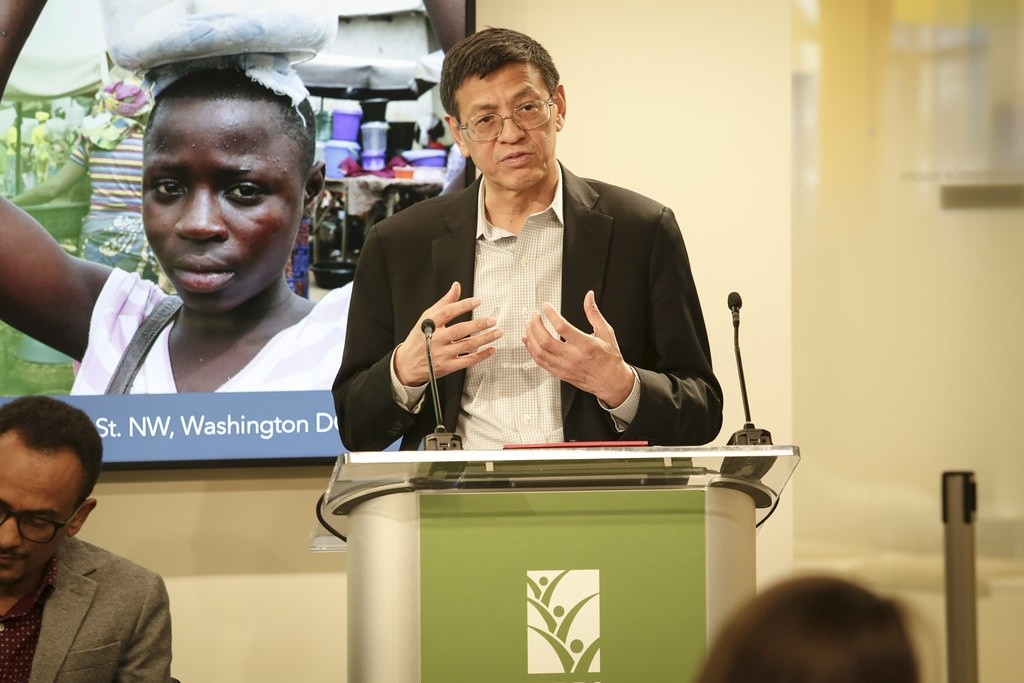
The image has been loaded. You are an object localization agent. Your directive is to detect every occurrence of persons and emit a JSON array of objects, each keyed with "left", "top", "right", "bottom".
[
  {"left": 0, "top": 395, "right": 173, "bottom": 683},
  {"left": 332, "top": 28, "right": 724, "bottom": 452},
  {"left": 0, "top": 0, "right": 353, "bottom": 396},
  {"left": 694, "top": 573, "right": 931, "bottom": 683}
]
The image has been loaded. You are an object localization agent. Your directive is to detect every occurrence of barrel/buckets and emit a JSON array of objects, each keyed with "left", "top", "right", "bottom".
[
  {"left": 324, "top": 139, "right": 361, "bottom": 179},
  {"left": 331, "top": 100, "right": 363, "bottom": 142},
  {"left": 395, "top": 167, "right": 415, "bottom": 181},
  {"left": 360, "top": 121, "right": 391, "bottom": 152},
  {"left": 361, "top": 152, "right": 386, "bottom": 172}
]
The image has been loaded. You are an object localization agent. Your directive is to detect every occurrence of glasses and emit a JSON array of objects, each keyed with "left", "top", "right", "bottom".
[
  {"left": 458, "top": 96, "right": 554, "bottom": 142},
  {"left": 0, "top": 495, "right": 86, "bottom": 544}
]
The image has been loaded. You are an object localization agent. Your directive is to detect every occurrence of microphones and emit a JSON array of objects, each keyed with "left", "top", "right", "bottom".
[
  {"left": 721, "top": 292, "right": 777, "bottom": 479},
  {"left": 418, "top": 319, "right": 467, "bottom": 484}
]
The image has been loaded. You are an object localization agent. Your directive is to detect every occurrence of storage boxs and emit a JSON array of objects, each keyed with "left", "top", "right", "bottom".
[{"left": 323, "top": 102, "right": 446, "bottom": 179}]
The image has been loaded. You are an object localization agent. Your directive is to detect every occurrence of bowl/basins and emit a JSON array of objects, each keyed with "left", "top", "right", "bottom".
[{"left": 310, "top": 262, "right": 356, "bottom": 289}]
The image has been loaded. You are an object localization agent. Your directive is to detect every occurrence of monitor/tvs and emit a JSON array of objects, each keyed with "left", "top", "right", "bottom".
[{"left": 0, "top": 0, "right": 476, "bottom": 469}]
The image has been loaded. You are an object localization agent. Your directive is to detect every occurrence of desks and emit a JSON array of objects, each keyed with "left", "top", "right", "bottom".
[{"left": 315, "top": 178, "right": 443, "bottom": 268}]
